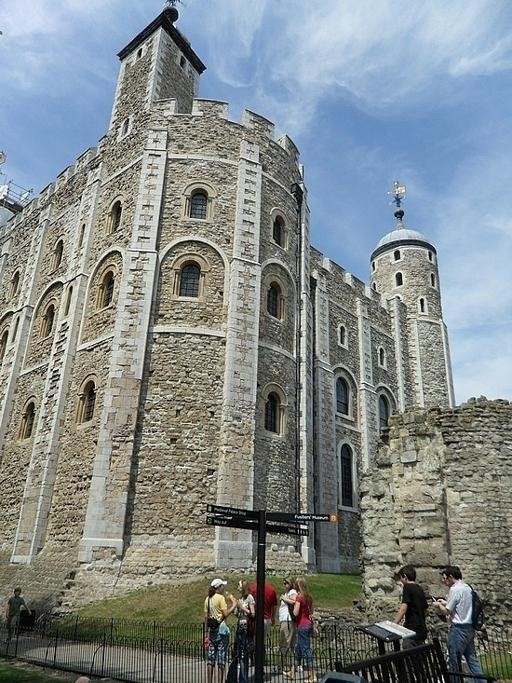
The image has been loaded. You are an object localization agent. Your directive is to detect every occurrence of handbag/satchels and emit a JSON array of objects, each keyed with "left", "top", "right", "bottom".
[
  {"left": 305, "top": 616, "right": 320, "bottom": 639},
  {"left": 246, "top": 608, "right": 256, "bottom": 636},
  {"left": 207, "top": 596, "right": 225, "bottom": 630},
  {"left": 236, "top": 608, "right": 249, "bottom": 635},
  {"left": 201, "top": 637, "right": 209, "bottom": 651},
  {"left": 217, "top": 622, "right": 231, "bottom": 638},
  {"left": 286, "top": 595, "right": 298, "bottom": 623}
]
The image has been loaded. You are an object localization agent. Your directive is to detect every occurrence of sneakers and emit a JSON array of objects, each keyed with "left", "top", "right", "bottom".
[
  {"left": 282, "top": 669, "right": 296, "bottom": 680},
  {"left": 304, "top": 676, "right": 318, "bottom": 683}
]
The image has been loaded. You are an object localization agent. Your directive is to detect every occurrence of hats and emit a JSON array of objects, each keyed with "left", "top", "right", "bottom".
[
  {"left": 283, "top": 576, "right": 294, "bottom": 587},
  {"left": 210, "top": 578, "right": 228, "bottom": 588}
]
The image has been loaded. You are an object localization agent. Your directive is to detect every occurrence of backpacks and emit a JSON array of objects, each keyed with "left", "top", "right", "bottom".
[{"left": 466, "top": 582, "right": 486, "bottom": 632}]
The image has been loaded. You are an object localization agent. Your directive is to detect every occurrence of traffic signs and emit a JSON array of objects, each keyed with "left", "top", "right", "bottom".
[{"left": 206, "top": 503, "right": 336, "bottom": 535}]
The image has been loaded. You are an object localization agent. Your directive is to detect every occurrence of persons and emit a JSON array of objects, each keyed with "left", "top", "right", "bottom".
[
  {"left": 436, "top": 570, "right": 453, "bottom": 637},
  {"left": 226, "top": 578, "right": 254, "bottom": 666},
  {"left": 249, "top": 573, "right": 276, "bottom": 665},
  {"left": 393, "top": 566, "right": 426, "bottom": 661},
  {"left": 284, "top": 576, "right": 318, "bottom": 682},
  {"left": 274, "top": 576, "right": 303, "bottom": 673},
  {"left": 432, "top": 565, "right": 487, "bottom": 682},
  {"left": 202, "top": 577, "right": 238, "bottom": 682},
  {"left": 7, "top": 587, "right": 34, "bottom": 640}
]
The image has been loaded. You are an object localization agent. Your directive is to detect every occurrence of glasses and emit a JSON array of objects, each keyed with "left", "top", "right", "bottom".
[{"left": 284, "top": 582, "right": 288, "bottom": 585}]
